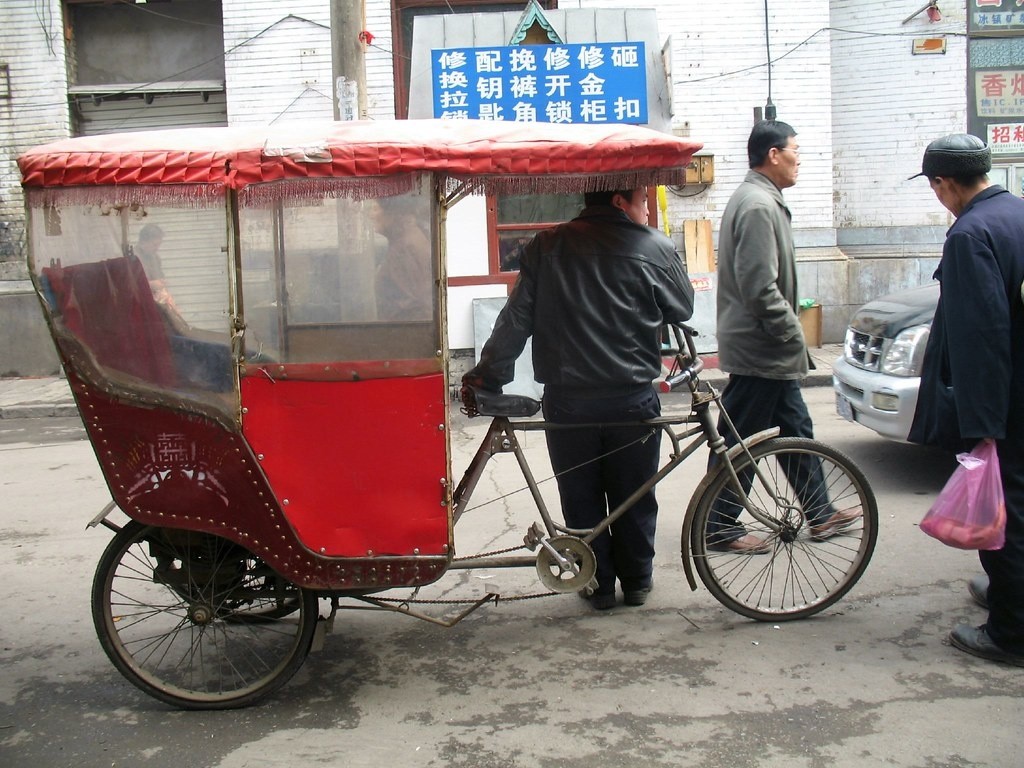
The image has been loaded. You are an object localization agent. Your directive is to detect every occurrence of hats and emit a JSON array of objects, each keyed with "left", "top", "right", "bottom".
[{"left": 906, "top": 133, "right": 992, "bottom": 182}]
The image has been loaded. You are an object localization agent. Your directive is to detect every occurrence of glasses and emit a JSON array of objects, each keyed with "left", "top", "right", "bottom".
[{"left": 778, "top": 147, "right": 803, "bottom": 159}]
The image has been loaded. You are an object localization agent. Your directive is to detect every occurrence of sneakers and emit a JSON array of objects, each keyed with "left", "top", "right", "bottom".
[
  {"left": 620, "top": 577, "right": 655, "bottom": 608},
  {"left": 576, "top": 578, "right": 618, "bottom": 614}
]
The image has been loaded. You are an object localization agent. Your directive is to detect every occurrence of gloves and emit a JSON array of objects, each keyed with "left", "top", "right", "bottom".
[{"left": 461, "top": 364, "right": 503, "bottom": 398}]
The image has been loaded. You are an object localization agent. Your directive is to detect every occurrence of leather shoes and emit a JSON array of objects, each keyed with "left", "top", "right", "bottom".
[
  {"left": 967, "top": 573, "right": 995, "bottom": 610},
  {"left": 703, "top": 531, "right": 774, "bottom": 555},
  {"left": 808, "top": 506, "right": 865, "bottom": 541},
  {"left": 947, "top": 623, "right": 1023, "bottom": 668}
]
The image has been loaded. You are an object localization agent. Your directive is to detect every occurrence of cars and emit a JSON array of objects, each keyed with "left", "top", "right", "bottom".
[{"left": 830, "top": 280, "right": 945, "bottom": 450}]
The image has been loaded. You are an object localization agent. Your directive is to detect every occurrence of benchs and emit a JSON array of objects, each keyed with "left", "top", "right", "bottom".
[{"left": 43, "top": 258, "right": 279, "bottom": 416}]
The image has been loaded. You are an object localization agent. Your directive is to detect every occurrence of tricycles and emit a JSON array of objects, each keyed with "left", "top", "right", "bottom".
[{"left": 13, "top": 117, "right": 881, "bottom": 710}]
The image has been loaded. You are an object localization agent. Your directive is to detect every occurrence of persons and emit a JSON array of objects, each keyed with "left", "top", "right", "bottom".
[
  {"left": 130, "top": 225, "right": 167, "bottom": 293},
  {"left": 906, "top": 135, "right": 1024, "bottom": 668},
  {"left": 462, "top": 180, "right": 695, "bottom": 609},
  {"left": 364, "top": 190, "right": 434, "bottom": 322},
  {"left": 705, "top": 121, "right": 864, "bottom": 554}
]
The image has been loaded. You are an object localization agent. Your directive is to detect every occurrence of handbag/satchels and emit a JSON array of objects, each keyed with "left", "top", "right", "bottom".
[{"left": 918, "top": 436, "right": 1011, "bottom": 551}]
